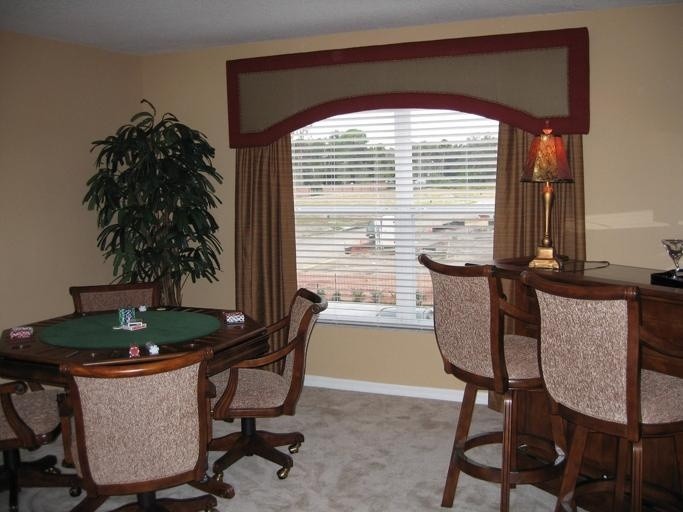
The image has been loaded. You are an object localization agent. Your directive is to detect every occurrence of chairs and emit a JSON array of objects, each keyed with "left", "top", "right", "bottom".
[
  {"left": 68, "top": 283, "right": 162, "bottom": 314},
  {"left": 208, "top": 287, "right": 327, "bottom": 482},
  {"left": 423, "top": 255, "right": 568, "bottom": 511},
  {"left": 1, "top": 381, "right": 82, "bottom": 511},
  {"left": 518, "top": 270, "right": 681, "bottom": 512},
  {"left": 55, "top": 348, "right": 235, "bottom": 512}
]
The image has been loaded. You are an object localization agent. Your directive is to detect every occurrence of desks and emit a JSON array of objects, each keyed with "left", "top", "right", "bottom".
[
  {"left": 1, "top": 307, "right": 267, "bottom": 390},
  {"left": 464, "top": 257, "right": 683, "bottom": 492}
]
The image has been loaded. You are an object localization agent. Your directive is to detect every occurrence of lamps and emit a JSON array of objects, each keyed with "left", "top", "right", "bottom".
[{"left": 520, "top": 118, "right": 576, "bottom": 271}]
